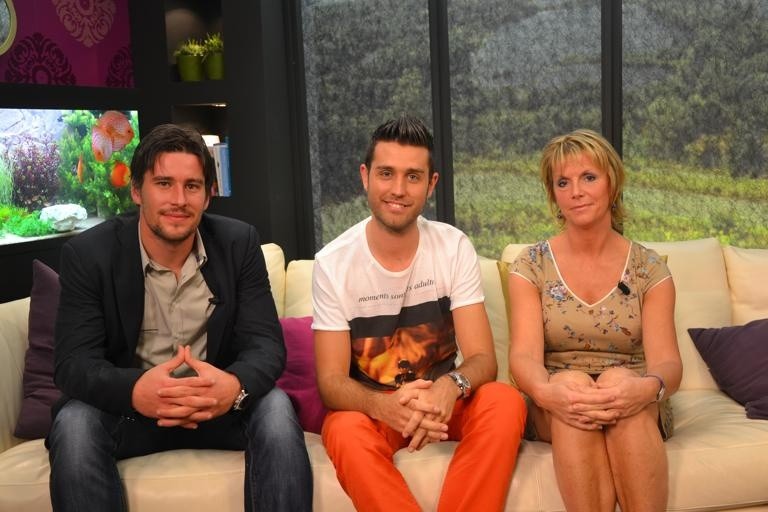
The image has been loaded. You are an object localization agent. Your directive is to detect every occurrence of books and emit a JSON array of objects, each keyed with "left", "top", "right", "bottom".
[{"left": 212, "top": 143, "right": 231, "bottom": 198}]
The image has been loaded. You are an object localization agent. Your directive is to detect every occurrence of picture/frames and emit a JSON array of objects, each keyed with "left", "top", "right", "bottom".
[{"left": 0, "top": 0, "right": 18, "bottom": 56}]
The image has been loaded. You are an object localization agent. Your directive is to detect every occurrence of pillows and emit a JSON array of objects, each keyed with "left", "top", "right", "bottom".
[
  {"left": 9, "top": 262, "right": 62, "bottom": 439},
  {"left": 285, "top": 253, "right": 509, "bottom": 385},
  {"left": 688, "top": 316, "right": 766, "bottom": 420},
  {"left": 279, "top": 317, "right": 322, "bottom": 435},
  {"left": 502, "top": 239, "right": 733, "bottom": 389},
  {"left": 261, "top": 242, "right": 285, "bottom": 316},
  {"left": 723, "top": 246, "right": 768, "bottom": 326}
]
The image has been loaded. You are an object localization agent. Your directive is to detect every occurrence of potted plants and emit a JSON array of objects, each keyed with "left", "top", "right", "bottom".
[
  {"left": 174, "top": 38, "right": 203, "bottom": 82},
  {"left": 201, "top": 30, "right": 225, "bottom": 82}
]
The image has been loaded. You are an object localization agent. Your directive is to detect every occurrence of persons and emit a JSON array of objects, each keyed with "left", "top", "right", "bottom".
[
  {"left": 311, "top": 115, "right": 529, "bottom": 512},
  {"left": 44, "top": 124, "right": 313, "bottom": 512},
  {"left": 507, "top": 130, "right": 683, "bottom": 512}
]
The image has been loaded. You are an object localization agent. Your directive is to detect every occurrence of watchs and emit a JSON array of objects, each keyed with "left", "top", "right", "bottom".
[
  {"left": 233, "top": 388, "right": 249, "bottom": 411},
  {"left": 645, "top": 374, "right": 665, "bottom": 406},
  {"left": 447, "top": 372, "right": 471, "bottom": 400}
]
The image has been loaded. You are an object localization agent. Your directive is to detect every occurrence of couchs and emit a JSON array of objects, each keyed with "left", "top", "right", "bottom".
[{"left": 0, "top": 237, "right": 768, "bottom": 512}]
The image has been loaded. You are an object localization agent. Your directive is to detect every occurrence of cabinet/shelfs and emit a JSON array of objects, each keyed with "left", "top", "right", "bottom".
[{"left": 0, "top": 236, "right": 71, "bottom": 303}]
